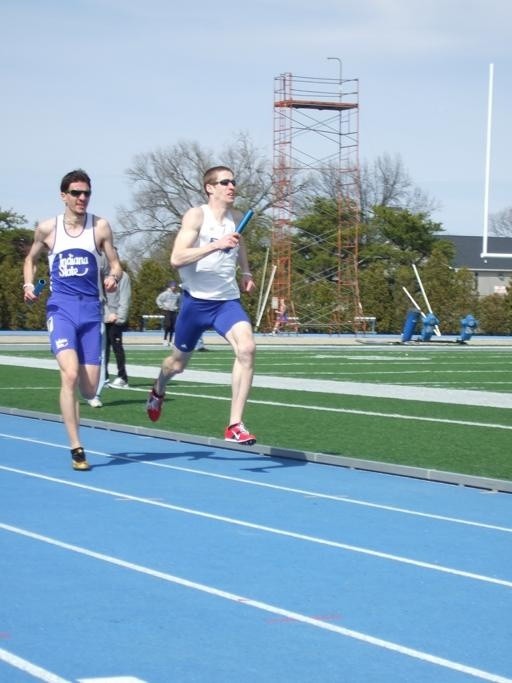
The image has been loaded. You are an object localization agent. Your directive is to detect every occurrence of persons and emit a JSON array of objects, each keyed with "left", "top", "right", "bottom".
[
  {"left": 99, "top": 246, "right": 132, "bottom": 390},
  {"left": 146, "top": 165, "right": 259, "bottom": 446},
  {"left": 22, "top": 167, "right": 128, "bottom": 470},
  {"left": 156, "top": 280, "right": 181, "bottom": 346},
  {"left": 271, "top": 298, "right": 298, "bottom": 334}
]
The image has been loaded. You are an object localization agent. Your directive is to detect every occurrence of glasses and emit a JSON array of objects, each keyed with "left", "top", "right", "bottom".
[
  {"left": 212, "top": 180, "right": 236, "bottom": 187},
  {"left": 66, "top": 191, "right": 90, "bottom": 197}
]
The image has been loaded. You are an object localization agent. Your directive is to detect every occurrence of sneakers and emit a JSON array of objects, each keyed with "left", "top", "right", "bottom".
[
  {"left": 87, "top": 398, "right": 103, "bottom": 407},
  {"left": 147, "top": 383, "right": 166, "bottom": 421},
  {"left": 225, "top": 422, "right": 256, "bottom": 445},
  {"left": 108, "top": 377, "right": 128, "bottom": 389},
  {"left": 71, "top": 447, "right": 89, "bottom": 470}
]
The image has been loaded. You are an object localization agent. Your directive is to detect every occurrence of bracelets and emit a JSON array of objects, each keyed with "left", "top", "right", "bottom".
[
  {"left": 23, "top": 282, "right": 35, "bottom": 289},
  {"left": 107, "top": 274, "right": 120, "bottom": 283},
  {"left": 239, "top": 271, "right": 254, "bottom": 278}
]
